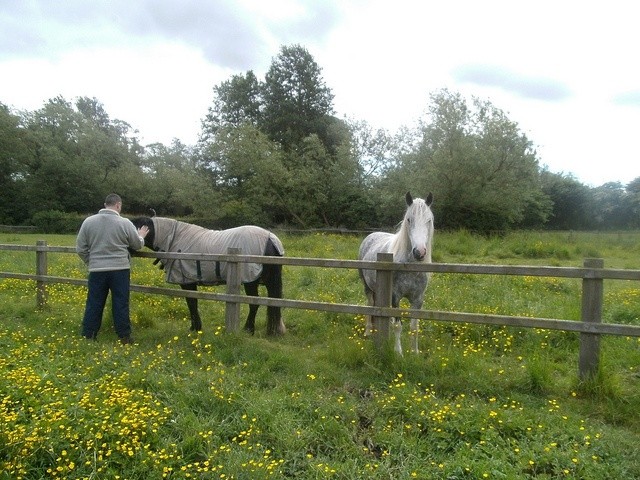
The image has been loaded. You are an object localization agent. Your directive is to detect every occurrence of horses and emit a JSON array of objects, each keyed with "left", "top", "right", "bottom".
[
  {"left": 357, "top": 192, "right": 435, "bottom": 357},
  {"left": 129, "top": 215, "right": 286, "bottom": 337}
]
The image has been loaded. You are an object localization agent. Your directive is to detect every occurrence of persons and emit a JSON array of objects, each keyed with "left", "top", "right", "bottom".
[{"left": 76, "top": 192, "right": 150, "bottom": 344}]
